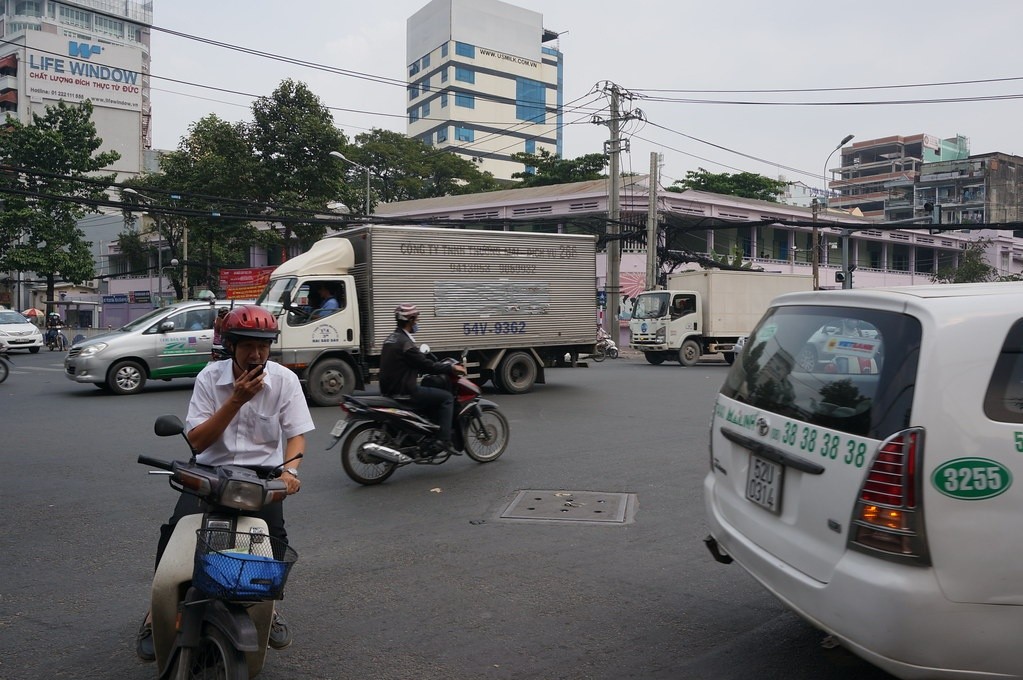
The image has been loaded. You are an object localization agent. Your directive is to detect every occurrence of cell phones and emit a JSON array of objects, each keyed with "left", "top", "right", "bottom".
[{"left": 248, "top": 362, "right": 264, "bottom": 382}]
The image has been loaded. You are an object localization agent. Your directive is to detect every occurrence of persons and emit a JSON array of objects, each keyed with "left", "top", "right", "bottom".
[
  {"left": 187, "top": 315, "right": 203, "bottom": 330},
  {"left": 379, "top": 304, "right": 468, "bottom": 456},
  {"left": 213, "top": 307, "right": 229, "bottom": 345},
  {"left": 299, "top": 283, "right": 338, "bottom": 319},
  {"left": 46, "top": 315, "right": 68, "bottom": 350},
  {"left": 597, "top": 323, "right": 609, "bottom": 341},
  {"left": 136, "top": 305, "right": 313, "bottom": 659}
]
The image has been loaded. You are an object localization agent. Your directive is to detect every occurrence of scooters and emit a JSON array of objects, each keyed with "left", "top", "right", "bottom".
[
  {"left": 590, "top": 331, "right": 620, "bottom": 361},
  {"left": 45, "top": 325, "right": 66, "bottom": 351},
  {"left": 0, "top": 348, "right": 15, "bottom": 383}
]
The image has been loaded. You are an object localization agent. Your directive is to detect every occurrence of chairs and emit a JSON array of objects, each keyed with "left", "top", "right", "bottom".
[
  {"left": 333, "top": 283, "right": 345, "bottom": 308},
  {"left": 685, "top": 300, "right": 695, "bottom": 310}
]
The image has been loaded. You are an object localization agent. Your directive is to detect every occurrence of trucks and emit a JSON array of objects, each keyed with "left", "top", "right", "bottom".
[
  {"left": 629, "top": 269, "right": 815, "bottom": 368},
  {"left": 256, "top": 225, "right": 601, "bottom": 408}
]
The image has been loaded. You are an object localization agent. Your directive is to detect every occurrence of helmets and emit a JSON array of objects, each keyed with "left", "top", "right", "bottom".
[
  {"left": 49, "top": 312, "right": 54, "bottom": 315},
  {"left": 221, "top": 305, "right": 280, "bottom": 338},
  {"left": 54, "top": 313, "right": 60, "bottom": 317},
  {"left": 395, "top": 303, "right": 420, "bottom": 320}
]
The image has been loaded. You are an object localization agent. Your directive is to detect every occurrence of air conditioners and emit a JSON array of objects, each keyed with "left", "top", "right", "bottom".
[
  {"left": 76, "top": 280, "right": 86, "bottom": 286},
  {"left": 24, "top": 277, "right": 34, "bottom": 285},
  {"left": 87, "top": 281, "right": 98, "bottom": 288}
]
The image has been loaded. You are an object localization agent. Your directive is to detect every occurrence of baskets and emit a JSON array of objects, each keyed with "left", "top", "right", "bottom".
[{"left": 192, "top": 529, "right": 298, "bottom": 602}]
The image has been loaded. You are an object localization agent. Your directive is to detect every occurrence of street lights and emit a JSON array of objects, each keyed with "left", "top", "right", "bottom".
[
  {"left": 122, "top": 186, "right": 163, "bottom": 305},
  {"left": 822, "top": 135, "right": 856, "bottom": 208},
  {"left": 329, "top": 151, "right": 370, "bottom": 214}
]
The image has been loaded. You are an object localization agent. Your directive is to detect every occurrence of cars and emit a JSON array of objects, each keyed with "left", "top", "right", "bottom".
[
  {"left": 62, "top": 291, "right": 298, "bottom": 395},
  {"left": 0, "top": 309, "right": 44, "bottom": 354},
  {"left": 701, "top": 280, "right": 1023, "bottom": 680}
]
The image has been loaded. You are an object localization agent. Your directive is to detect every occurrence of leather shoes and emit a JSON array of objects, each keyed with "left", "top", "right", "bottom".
[{"left": 435, "top": 441, "right": 463, "bottom": 455}]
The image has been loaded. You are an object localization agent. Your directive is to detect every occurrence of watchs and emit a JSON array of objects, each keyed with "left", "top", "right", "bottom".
[{"left": 283, "top": 468, "right": 298, "bottom": 477}]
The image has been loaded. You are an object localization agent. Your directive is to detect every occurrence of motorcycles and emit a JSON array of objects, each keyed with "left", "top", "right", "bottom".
[
  {"left": 322, "top": 341, "right": 512, "bottom": 484},
  {"left": 133, "top": 413, "right": 302, "bottom": 680}
]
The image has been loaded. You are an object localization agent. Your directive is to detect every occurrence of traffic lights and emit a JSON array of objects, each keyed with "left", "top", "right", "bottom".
[{"left": 834, "top": 270, "right": 847, "bottom": 282}]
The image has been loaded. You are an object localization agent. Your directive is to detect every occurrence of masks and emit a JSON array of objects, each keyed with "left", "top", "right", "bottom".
[
  {"left": 411, "top": 322, "right": 418, "bottom": 334},
  {"left": 54, "top": 316, "right": 57, "bottom": 319}
]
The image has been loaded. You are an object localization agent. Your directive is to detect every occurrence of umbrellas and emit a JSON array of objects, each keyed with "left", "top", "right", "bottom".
[{"left": 21, "top": 307, "right": 44, "bottom": 318}]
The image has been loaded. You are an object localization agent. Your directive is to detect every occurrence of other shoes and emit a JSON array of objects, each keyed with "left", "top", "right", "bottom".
[
  {"left": 268, "top": 608, "right": 292, "bottom": 650},
  {"left": 135, "top": 610, "right": 155, "bottom": 663}
]
